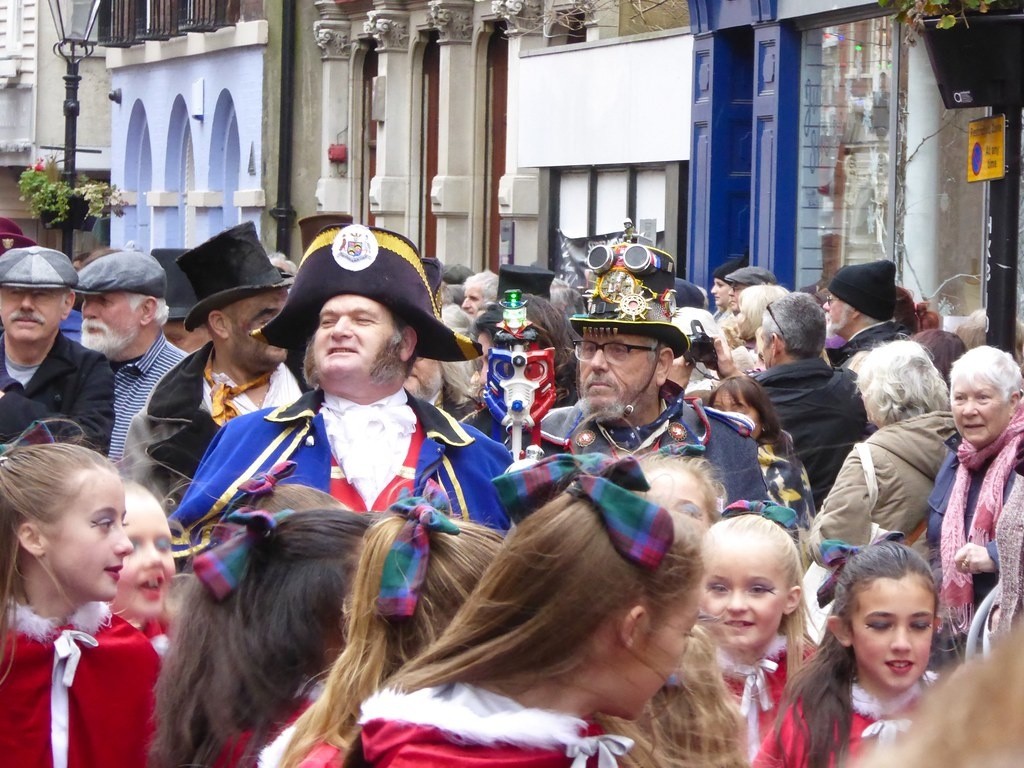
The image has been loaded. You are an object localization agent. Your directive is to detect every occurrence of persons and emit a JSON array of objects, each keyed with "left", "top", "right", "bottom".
[{"left": 0, "top": 220, "right": 1024, "bottom": 768}]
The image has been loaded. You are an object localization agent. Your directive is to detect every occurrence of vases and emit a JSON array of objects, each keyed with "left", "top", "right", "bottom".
[
  {"left": 40, "top": 195, "right": 99, "bottom": 231},
  {"left": 924, "top": 15, "right": 1024, "bottom": 110}
]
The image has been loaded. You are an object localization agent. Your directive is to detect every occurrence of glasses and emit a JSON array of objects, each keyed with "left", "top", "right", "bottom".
[
  {"left": 7, "top": 287, "right": 53, "bottom": 301},
  {"left": 826, "top": 296, "right": 840, "bottom": 304},
  {"left": 573, "top": 340, "right": 659, "bottom": 364},
  {"left": 733, "top": 286, "right": 743, "bottom": 292},
  {"left": 766, "top": 301, "right": 784, "bottom": 335},
  {"left": 587, "top": 244, "right": 652, "bottom": 274}
]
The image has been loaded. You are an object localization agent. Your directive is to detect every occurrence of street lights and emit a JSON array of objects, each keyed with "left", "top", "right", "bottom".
[{"left": 39, "top": 0, "right": 102, "bottom": 261}]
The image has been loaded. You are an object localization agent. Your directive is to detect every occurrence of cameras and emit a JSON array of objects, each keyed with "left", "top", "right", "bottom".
[{"left": 682, "top": 319, "right": 717, "bottom": 362}]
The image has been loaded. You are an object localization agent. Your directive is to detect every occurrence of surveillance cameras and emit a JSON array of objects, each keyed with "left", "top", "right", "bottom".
[{"left": 108, "top": 88, "right": 121, "bottom": 104}]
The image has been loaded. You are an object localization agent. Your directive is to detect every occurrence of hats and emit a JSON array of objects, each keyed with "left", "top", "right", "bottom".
[
  {"left": 828, "top": 259, "right": 896, "bottom": 321},
  {"left": 72, "top": 252, "right": 168, "bottom": 298},
  {"left": 443, "top": 263, "right": 476, "bottom": 284},
  {"left": 0, "top": 245, "right": 78, "bottom": 289},
  {"left": 249, "top": 223, "right": 485, "bottom": 362},
  {"left": 724, "top": 266, "right": 776, "bottom": 285},
  {"left": 712, "top": 256, "right": 749, "bottom": 281},
  {"left": 0, "top": 217, "right": 37, "bottom": 255},
  {"left": 174, "top": 220, "right": 295, "bottom": 333},
  {"left": 151, "top": 248, "right": 199, "bottom": 321},
  {"left": 494, "top": 264, "right": 556, "bottom": 303},
  {"left": 570, "top": 217, "right": 690, "bottom": 360}
]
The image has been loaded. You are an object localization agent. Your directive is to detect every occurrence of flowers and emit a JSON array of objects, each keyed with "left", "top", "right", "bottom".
[{"left": 16, "top": 154, "right": 130, "bottom": 229}]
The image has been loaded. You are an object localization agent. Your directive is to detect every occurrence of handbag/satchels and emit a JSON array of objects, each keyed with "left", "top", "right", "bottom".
[{"left": 801, "top": 522, "right": 905, "bottom": 645}]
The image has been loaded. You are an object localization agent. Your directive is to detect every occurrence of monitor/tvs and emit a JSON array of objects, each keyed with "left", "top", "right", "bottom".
[{"left": 919, "top": 12, "right": 1024, "bottom": 110}]
